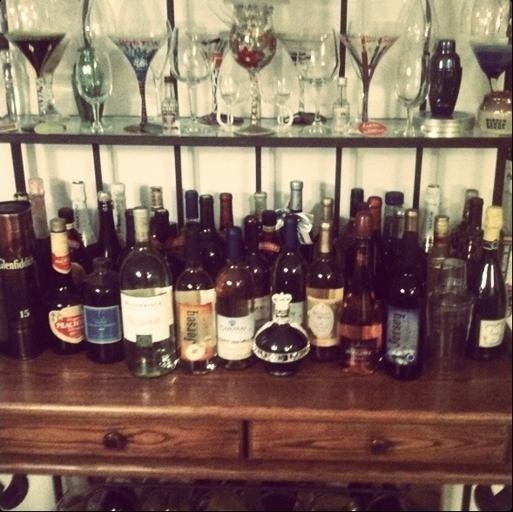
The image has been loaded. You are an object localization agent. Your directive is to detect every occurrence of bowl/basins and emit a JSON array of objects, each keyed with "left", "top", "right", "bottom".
[{"left": 477, "top": 91, "right": 512, "bottom": 135}]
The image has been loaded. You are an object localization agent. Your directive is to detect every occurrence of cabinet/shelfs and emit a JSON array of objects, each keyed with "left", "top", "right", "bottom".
[{"left": 1, "top": 116, "right": 513, "bottom": 510}]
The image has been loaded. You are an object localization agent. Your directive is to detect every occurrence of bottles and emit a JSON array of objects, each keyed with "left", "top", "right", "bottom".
[
  {"left": 0, "top": 178, "right": 513, "bottom": 377},
  {"left": 425, "top": 36, "right": 463, "bottom": 119}
]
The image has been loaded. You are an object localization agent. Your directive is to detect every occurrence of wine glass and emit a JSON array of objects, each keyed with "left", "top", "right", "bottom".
[
  {"left": 468, "top": 34, "right": 512, "bottom": 92},
  {"left": 0, "top": 0, "right": 432, "bottom": 136}
]
